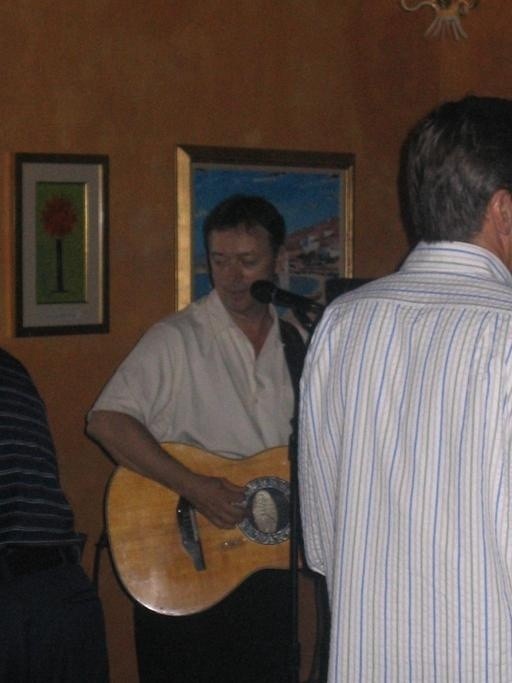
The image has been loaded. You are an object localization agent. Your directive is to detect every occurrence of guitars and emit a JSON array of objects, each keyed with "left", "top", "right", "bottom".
[{"left": 104, "top": 443, "right": 305, "bottom": 616}]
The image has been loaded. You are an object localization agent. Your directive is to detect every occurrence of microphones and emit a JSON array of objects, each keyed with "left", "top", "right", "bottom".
[{"left": 250, "top": 279, "right": 325, "bottom": 314}]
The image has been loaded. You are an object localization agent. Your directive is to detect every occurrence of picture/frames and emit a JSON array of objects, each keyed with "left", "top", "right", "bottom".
[
  {"left": 174, "top": 145, "right": 356, "bottom": 345},
  {"left": 15, "top": 153, "right": 109, "bottom": 336}
]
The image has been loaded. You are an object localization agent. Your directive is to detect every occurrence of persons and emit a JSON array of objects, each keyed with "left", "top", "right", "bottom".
[
  {"left": 0, "top": 347, "right": 109, "bottom": 683},
  {"left": 82, "top": 193, "right": 318, "bottom": 681},
  {"left": 296, "top": 94, "right": 512, "bottom": 682}
]
